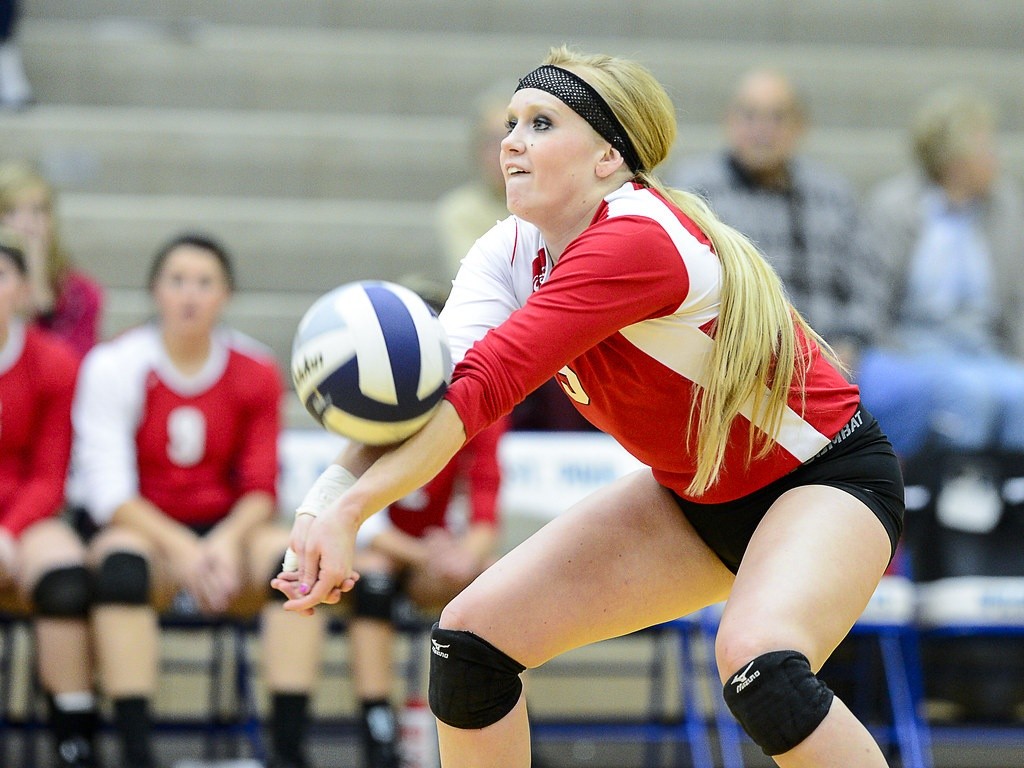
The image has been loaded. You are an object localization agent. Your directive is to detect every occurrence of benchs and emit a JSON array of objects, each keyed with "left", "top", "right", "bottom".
[{"left": 0, "top": 1, "right": 1024, "bottom": 447}]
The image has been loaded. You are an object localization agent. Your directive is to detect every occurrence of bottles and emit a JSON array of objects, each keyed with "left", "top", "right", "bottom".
[{"left": 396, "top": 698, "right": 440, "bottom": 767}]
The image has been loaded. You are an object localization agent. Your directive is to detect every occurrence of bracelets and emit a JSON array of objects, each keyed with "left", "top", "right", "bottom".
[{"left": 294, "top": 463, "right": 358, "bottom": 519}]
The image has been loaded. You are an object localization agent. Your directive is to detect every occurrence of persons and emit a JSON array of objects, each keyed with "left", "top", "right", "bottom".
[
  {"left": 872, "top": 99, "right": 1013, "bottom": 355},
  {"left": 272, "top": 41, "right": 906, "bottom": 766},
  {"left": 433, "top": 87, "right": 535, "bottom": 297},
  {"left": 65, "top": 234, "right": 323, "bottom": 767},
  {"left": 0, "top": 245, "right": 111, "bottom": 763},
  {"left": 337, "top": 401, "right": 502, "bottom": 763},
  {"left": 0, "top": 158, "right": 100, "bottom": 359},
  {"left": 680, "top": 68, "right": 876, "bottom": 395}
]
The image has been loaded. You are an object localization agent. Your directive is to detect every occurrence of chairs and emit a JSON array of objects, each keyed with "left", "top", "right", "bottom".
[{"left": 1, "top": 515, "right": 1023, "bottom": 768}]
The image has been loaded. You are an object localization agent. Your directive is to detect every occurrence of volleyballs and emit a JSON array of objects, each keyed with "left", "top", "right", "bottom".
[{"left": 291, "top": 280, "right": 449, "bottom": 444}]
